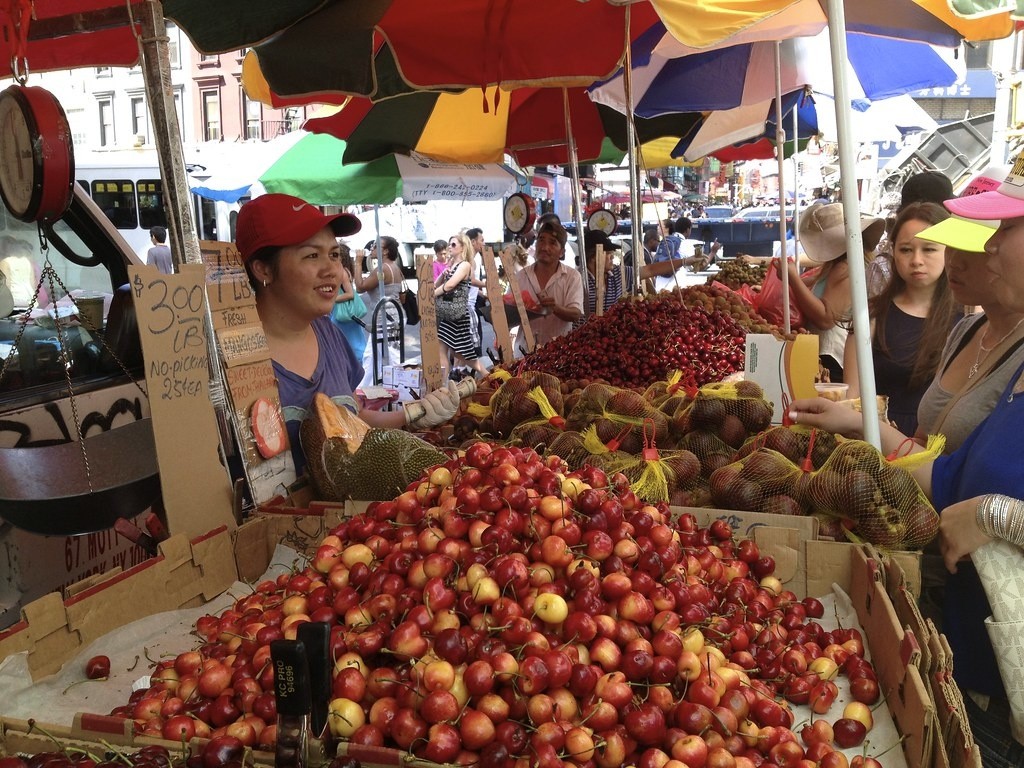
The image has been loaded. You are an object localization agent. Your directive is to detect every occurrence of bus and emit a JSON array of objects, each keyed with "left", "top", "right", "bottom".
[{"left": 0, "top": 165, "right": 244, "bottom": 294}]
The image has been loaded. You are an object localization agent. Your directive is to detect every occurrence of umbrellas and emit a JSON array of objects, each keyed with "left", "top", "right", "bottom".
[{"left": 0, "top": 0, "right": 1024, "bottom": 483}]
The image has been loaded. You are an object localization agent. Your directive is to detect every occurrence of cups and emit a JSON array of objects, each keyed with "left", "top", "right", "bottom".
[
  {"left": 74, "top": 295, "right": 105, "bottom": 331},
  {"left": 815, "top": 382, "right": 850, "bottom": 402}
]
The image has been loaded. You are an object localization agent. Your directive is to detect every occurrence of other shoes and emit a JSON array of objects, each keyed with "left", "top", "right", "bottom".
[
  {"left": 461, "top": 367, "right": 476, "bottom": 379},
  {"left": 449, "top": 369, "right": 462, "bottom": 383}
]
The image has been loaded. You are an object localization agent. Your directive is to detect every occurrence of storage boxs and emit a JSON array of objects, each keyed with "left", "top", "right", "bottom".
[
  {"left": 382, "top": 362, "right": 446, "bottom": 412},
  {"left": 1, "top": 474, "right": 983, "bottom": 768}
]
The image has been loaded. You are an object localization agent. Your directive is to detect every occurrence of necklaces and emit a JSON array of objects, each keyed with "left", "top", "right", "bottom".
[{"left": 968, "top": 317, "right": 1024, "bottom": 379}]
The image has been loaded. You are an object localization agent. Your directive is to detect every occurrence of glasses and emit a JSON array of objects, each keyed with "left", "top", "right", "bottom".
[
  {"left": 657, "top": 226, "right": 667, "bottom": 229},
  {"left": 450, "top": 243, "right": 462, "bottom": 248},
  {"left": 371, "top": 244, "right": 386, "bottom": 249}
]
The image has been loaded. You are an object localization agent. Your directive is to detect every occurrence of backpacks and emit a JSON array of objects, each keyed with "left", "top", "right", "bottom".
[{"left": 656, "top": 233, "right": 685, "bottom": 258}]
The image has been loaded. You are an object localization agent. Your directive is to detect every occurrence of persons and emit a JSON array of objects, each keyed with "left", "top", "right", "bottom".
[
  {"left": 500, "top": 198, "right": 1023, "bottom": 525},
  {"left": 147, "top": 226, "right": 172, "bottom": 275},
  {"left": 234, "top": 195, "right": 461, "bottom": 485},
  {"left": 325, "top": 240, "right": 367, "bottom": 366},
  {"left": 574, "top": 229, "right": 711, "bottom": 333},
  {"left": 356, "top": 235, "right": 407, "bottom": 388},
  {"left": 788, "top": 143, "right": 1024, "bottom": 729},
  {"left": 621, "top": 230, "right": 661, "bottom": 268},
  {"left": 0, "top": 269, "right": 16, "bottom": 321},
  {"left": 0, "top": 235, "right": 40, "bottom": 308},
  {"left": 433, "top": 240, "right": 450, "bottom": 283},
  {"left": 434, "top": 233, "right": 490, "bottom": 386},
  {"left": 657, "top": 219, "right": 674, "bottom": 239},
  {"left": 448, "top": 226, "right": 487, "bottom": 382},
  {"left": 504, "top": 222, "right": 583, "bottom": 360},
  {"left": 656, "top": 217, "right": 723, "bottom": 294},
  {"left": 16, "top": 240, "right": 48, "bottom": 308}
]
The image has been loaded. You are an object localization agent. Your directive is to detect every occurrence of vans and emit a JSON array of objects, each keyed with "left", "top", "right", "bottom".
[{"left": 733, "top": 205, "right": 808, "bottom": 220}]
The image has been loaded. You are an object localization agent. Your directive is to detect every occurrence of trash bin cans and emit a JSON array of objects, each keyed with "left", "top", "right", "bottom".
[{"left": 75, "top": 295, "right": 105, "bottom": 331}]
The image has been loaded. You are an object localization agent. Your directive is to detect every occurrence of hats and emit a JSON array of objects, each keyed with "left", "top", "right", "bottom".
[
  {"left": 914, "top": 164, "right": 1015, "bottom": 253},
  {"left": 235, "top": 193, "right": 362, "bottom": 264},
  {"left": 584, "top": 229, "right": 622, "bottom": 251},
  {"left": 942, "top": 148, "right": 1024, "bottom": 220},
  {"left": 798, "top": 202, "right": 886, "bottom": 263},
  {"left": 538, "top": 221, "right": 567, "bottom": 260},
  {"left": 896, "top": 172, "right": 960, "bottom": 213}
]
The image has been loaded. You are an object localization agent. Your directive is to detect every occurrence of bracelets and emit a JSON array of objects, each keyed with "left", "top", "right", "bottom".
[{"left": 975, "top": 493, "right": 1024, "bottom": 547}]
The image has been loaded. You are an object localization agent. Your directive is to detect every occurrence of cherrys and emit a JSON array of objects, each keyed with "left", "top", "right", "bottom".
[
  {"left": 490, "top": 297, "right": 751, "bottom": 390},
  {"left": 85, "top": 443, "right": 879, "bottom": 768}
]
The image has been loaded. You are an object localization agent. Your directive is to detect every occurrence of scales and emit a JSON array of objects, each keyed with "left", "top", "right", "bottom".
[
  {"left": 589, "top": 210, "right": 619, "bottom": 239},
  {"left": 479, "top": 186, "right": 547, "bottom": 337},
  {"left": 1, "top": 1, "right": 164, "bottom": 538}
]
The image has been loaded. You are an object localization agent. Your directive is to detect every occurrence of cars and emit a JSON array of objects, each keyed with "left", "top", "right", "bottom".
[
  {"left": 1, "top": 175, "right": 159, "bottom": 455},
  {"left": 705, "top": 205, "right": 737, "bottom": 220}
]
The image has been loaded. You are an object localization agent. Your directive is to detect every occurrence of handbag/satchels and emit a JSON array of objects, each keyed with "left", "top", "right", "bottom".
[
  {"left": 335, "top": 268, "right": 368, "bottom": 323},
  {"left": 968, "top": 536, "right": 1024, "bottom": 746},
  {"left": 435, "top": 260, "right": 472, "bottom": 321},
  {"left": 399, "top": 270, "right": 421, "bottom": 326}
]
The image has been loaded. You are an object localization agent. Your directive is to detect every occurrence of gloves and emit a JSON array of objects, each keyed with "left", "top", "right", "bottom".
[
  {"left": 411, "top": 379, "right": 461, "bottom": 429},
  {"left": 682, "top": 254, "right": 710, "bottom": 266}
]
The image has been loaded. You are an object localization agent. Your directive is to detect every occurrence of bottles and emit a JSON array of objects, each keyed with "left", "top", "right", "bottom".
[{"left": 350, "top": 250, "right": 371, "bottom": 258}]
